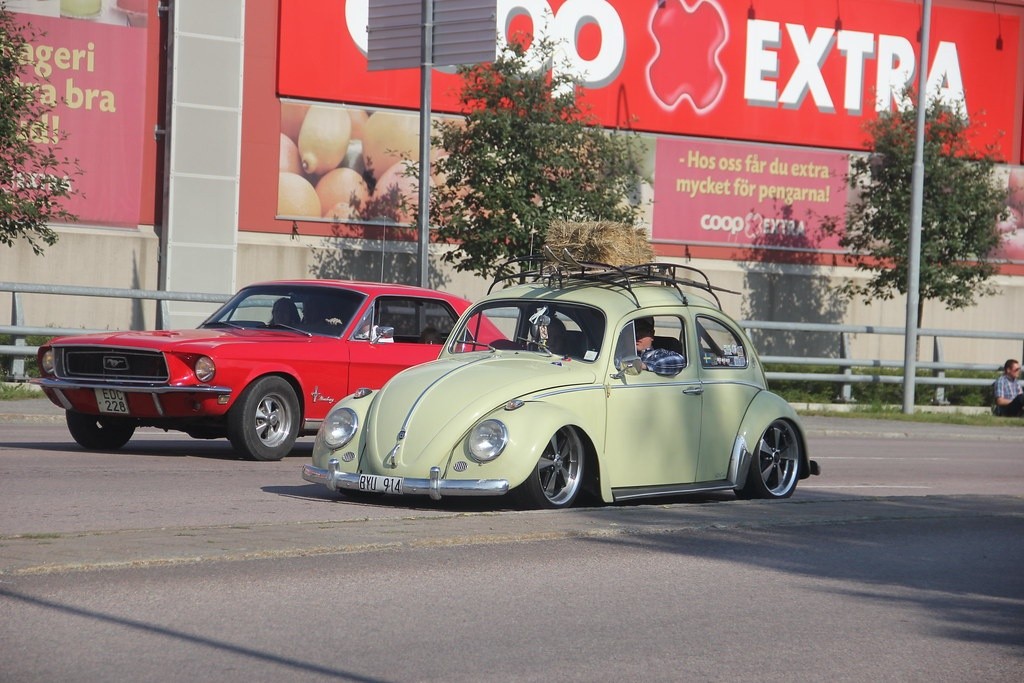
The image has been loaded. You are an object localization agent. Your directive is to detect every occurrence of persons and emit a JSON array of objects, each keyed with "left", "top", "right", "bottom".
[
  {"left": 268, "top": 298, "right": 300, "bottom": 327},
  {"left": 615, "top": 315, "right": 684, "bottom": 378},
  {"left": 419, "top": 328, "right": 443, "bottom": 344},
  {"left": 325, "top": 294, "right": 383, "bottom": 339},
  {"left": 991, "top": 359, "right": 1024, "bottom": 417}
]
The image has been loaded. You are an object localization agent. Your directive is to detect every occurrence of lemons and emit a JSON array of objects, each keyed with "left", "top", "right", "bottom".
[{"left": 278, "top": 101, "right": 471, "bottom": 242}]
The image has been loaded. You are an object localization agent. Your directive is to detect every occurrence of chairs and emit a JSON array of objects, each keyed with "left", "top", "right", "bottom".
[
  {"left": 565, "top": 329, "right": 589, "bottom": 356},
  {"left": 654, "top": 335, "right": 682, "bottom": 354}
]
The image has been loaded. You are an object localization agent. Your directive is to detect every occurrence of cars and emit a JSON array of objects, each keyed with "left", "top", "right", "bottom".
[
  {"left": 301, "top": 254, "right": 824, "bottom": 515},
  {"left": 27, "top": 277, "right": 515, "bottom": 463}
]
